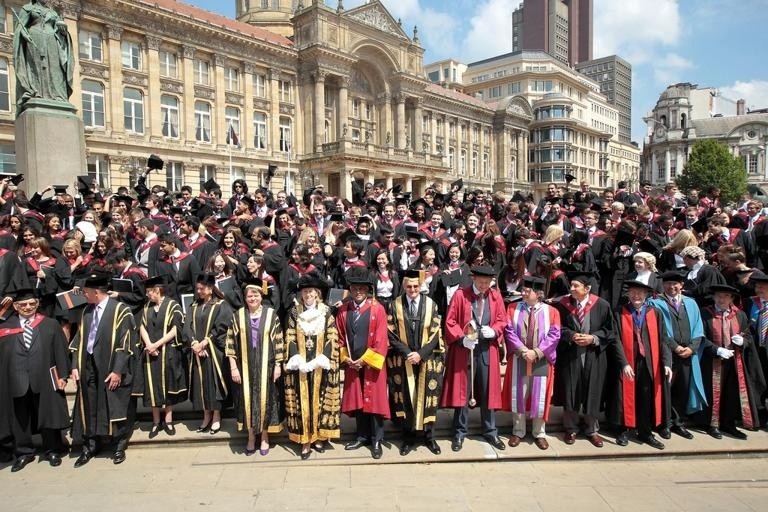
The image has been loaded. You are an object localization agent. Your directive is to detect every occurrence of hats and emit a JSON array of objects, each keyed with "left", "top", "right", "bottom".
[
  {"left": 386, "top": 184, "right": 402, "bottom": 194},
  {"left": 416, "top": 239, "right": 437, "bottom": 252},
  {"left": 347, "top": 278, "right": 373, "bottom": 284},
  {"left": 267, "top": 163, "right": 277, "bottom": 177},
  {"left": 470, "top": 266, "right": 496, "bottom": 277},
  {"left": 239, "top": 278, "right": 263, "bottom": 288},
  {"left": 240, "top": 196, "right": 255, "bottom": 208},
  {"left": 148, "top": 154, "right": 164, "bottom": 170},
  {"left": 202, "top": 176, "right": 220, "bottom": 194},
  {"left": 563, "top": 173, "right": 577, "bottom": 181},
  {"left": 707, "top": 285, "right": 740, "bottom": 294},
  {"left": 567, "top": 271, "right": 592, "bottom": 285},
  {"left": 592, "top": 199, "right": 604, "bottom": 211},
  {"left": 2, "top": 288, "right": 41, "bottom": 302},
  {"left": 521, "top": 276, "right": 547, "bottom": 291},
  {"left": 113, "top": 195, "right": 134, "bottom": 205},
  {"left": 287, "top": 192, "right": 297, "bottom": 208},
  {"left": 297, "top": 280, "right": 327, "bottom": 290},
  {"left": 359, "top": 216, "right": 371, "bottom": 222},
  {"left": 274, "top": 209, "right": 288, "bottom": 217},
  {"left": 7, "top": 173, "right": 25, "bottom": 186},
  {"left": 139, "top": 276, "right": 166, "bottom": 287},
  {"left": 84, "top": 271, "right": 112, "bottom": 289},
  {"left": 749, "top": 276, "right": 768, "bottom": 283},
  {"left": 395, "top": 198, "right": 409, "bottom": 207},
  {"left": 192, "top": 272, "right": 218, "bottom": 284},
  {"left": 169, "top": 207, "right": 187, "bottom": 214},
  {"left": 52, "top": 185, "right": 69, "bottom": 193},
  {"left": 623, "top": 279, "right": 653, "bottom": 289},
  {"left": 398, "top": 270, "right": 419, "bottom": 278},
  {"left": 410, "top": 198, "right": 431, "bottom": 209},
  {"left": 544, "top": 196, "right": 564, "bottom": 205},
  {"left": 78, "top": 175, "right": 95, "bottom": 196},
  {"left": 366, "top": 199, "right": 383, "bottom": 212},
  {"left": 451, "top": 178, "right": 463, "bottom": 192},
  {"left": 404, "top": 230, "right": 426, "bottom": 239},
  {"left": 656, "top": 271, "right": 684, "bottom": 281},
  {"left": 330, "top": 213, "right": 345, "bottom": 221}
]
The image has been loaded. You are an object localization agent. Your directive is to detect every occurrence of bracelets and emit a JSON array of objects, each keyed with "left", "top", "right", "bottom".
[{"left": 228, "top": 366, "right": 237, "bottom": 370}]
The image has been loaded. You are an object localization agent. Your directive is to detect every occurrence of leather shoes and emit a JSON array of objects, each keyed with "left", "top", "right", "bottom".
[
  {"left": 482, "top": 434, "right": 506, "bottom": 451},
  {"left": 508, "top": 434, "right": 521, "bottom": 447},
  {"left": 534, "top": 437, "right": 549, "bottom": 450},
  {"left": 615, "top": 429, "right": 629, "bottom": 447},
  {"left": 636, "top": 432, "right": 665, "bottom": 450},
  {"left": 590, "top": 435, "right": 603, "bottom": 447},
  {"left": 112, "top": 449, "right": 126, "bottom": 464},
  {"left": 370, "top": 440, "right": 383, "bottom": 459},
  {"left": 658, "top": 425, "right": 671, "bottom": 439},
  {"left": 726, "top": 429, "right": 747, "bottom": 440},
  {"left": 565, "top": 431, "right": 576, "bottom": 445},
  {"left": 47, "top": 451, "right": 63, "bottom": 467},
  {"left": 424, "top": 440, "right": 441, "bottom": 455},
  {"left": 11, "top": 453, "right": 35, "bottom": 472},
  {"left": 399, "top": 441, "right": 411, "bottom": 456},
  {"left": 344, "top": 437, "right": 365, "bottom": 450},
  {"left": 671, "top": 424, "right": 694, "bottom": 440},
  {"left": 707, "top": 427, "right": 722, "bottom": 439},
  {"left": 73, "top": 452, "right": 94, "bottom": 468},
  {"left": 451, "top": 434, "right": 462, "bottom": 451}
]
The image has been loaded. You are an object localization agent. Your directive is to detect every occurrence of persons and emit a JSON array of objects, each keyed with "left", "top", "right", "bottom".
[
  {"left": 241, "top": 254, "right": 280, "bottom": 313},
  {"left": 21, "top": 217, "right": 44, "bottom": 245},
  {"left": 55, "top": 239, "right": 95, "bottom": 343},
  {"left": 10, "top": 0, "right": 75, "bottom": 101},
  {"left": 286, "top": 227, "right": 446, "bottom": 272},
  {"left": 178, "top": 217, "right": 217, "bottom": 266},
  {"left": 153, "top": 232, "right": 203, "bottom": 306},
  {"left": 548, "top": 270, "right": 617, "bottom": 447},
  {"left": 700, "top": 285, "right": 760, "bottom": 439},
  {"left": 749, "top": 271, "right": 768, "bottom": 432},
  {"left": 644, "top": 272, "right": 709, "bottom": 440},
  {"left": 1, "top": 154, "right": 768, "bottom": 233},
  {"left": 504, "top": 276, "right": 562, "bottom": 450},
  {"left": 106, "top": 246, "right": 151, "bottom": 329},
  {"left": 8, "top": 212, "right": 24, "bottom": 238},
  {"left": 41, "top": 212, "right": 70, "bottom": 252},
  {"left": 224, "top": 278, "right": 285, "bottom": 456},
  {"left": 181, "top": 272, "right": 232, "bottom": 434},
  {"left": 704, "top": 214, "right": 757, "bottom": 271},
  {"left": 385, "top": 268, "right": 446, "bottom": 455},
  {"left": 335, "top": 264, "right": 390, "bottom": 458},
  {"left": 211, "top": 228, "right": 289, "bottom": 273},
  {"left": 510, "top": 226, "right": 615, "bottom": 279},
  {"left": 436, "top": 220, "right": 507, "bottom": 268},
  {"left": 67, "top": 270, "right": 142, "bottom": 469},
  {"left": 444, "top": 263, "right": 506, "bottom": 450},
  {"left": 606, "top": 281, "right": 672, "bottom": 449},
  {"left": 611, "top": 220, "right": 707, "bottom": 275},
  {"left": 0, "top": 288, "right": 72, "bottom": 474},
  {"left": 1, "top": 236, "right": 56, "bottom": 327},
  {"left": 137, "top": 276, "right": 188, "bottom": 438},
  {"left": 285, "top": 275, "right": 340, "bottom": 461}
]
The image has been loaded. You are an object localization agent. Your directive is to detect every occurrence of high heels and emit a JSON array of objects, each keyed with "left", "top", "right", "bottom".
[
  {"left": 197, "top": 417, "right": 211, "bottom": 432},
  {"left": 245, "top": 440, "right": 256, "bottom": 456},
  {"left": 210, "top": 417, "right": 222, "bottom": 435},
  {"left": 148, "top": 423, "right": 161, "bottom": 439},
  {"left": 313, "top": 444, "right": 324, "bottom": 453},
  {"left": 260, "top": 432, "right": 269, "bottom": 455},
  {"left": 301, "top": 448, "right": 311, "bottom": 460},
  {"left": 161, "top": 419, "right": 176, "bottom": 435}
]
